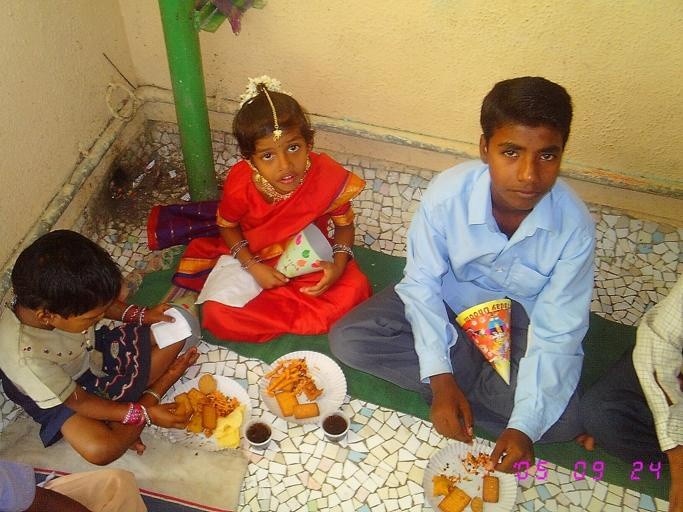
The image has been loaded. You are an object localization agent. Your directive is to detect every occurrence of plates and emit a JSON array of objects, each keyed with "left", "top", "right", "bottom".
[
  {"left": 422, "top": 441, "right": 517, "bottom": 512},
  {"left": 159, "top": 372, "right": 251, "bottom": 452},
  {"left": 258, "top": 348, "right": 346, "bottom": 426}
]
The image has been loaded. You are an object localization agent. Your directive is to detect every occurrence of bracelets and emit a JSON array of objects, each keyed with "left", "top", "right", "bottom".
[
  {"left": 241, "top": 256, "right": 264, "bottom": 272},
  {"left": 330, "top": 244, "right": 355, "bottom": 260},
  {"left": 143, "top": 389, "right": 162, "bottom": 403},
  {"left": 120, "top": 303, "right": 151, "bottom": 328},
  {"left": 124, "top": 400, "right": 153, "bottom": 430},
  {"left": 228, "top": 239, "right": 249, "bottom": 257}
]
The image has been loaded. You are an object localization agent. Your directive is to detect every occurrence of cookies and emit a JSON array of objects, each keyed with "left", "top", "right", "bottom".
[
  {"left": 275, "top": 390, "right": 321, "bottom": 420},
  {"left": 173, "top": 373, "right": 218, "bottom": 440},
  {"left": 431, "top": 471, "right": 500, "bottom": 512}
]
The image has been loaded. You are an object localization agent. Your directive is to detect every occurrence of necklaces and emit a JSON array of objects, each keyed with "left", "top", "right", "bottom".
[{"left": 254, "top": 172, "right": 307, "bottom": 205}]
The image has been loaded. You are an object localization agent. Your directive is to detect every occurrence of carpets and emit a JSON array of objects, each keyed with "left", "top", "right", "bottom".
[
  {"left": 0, "top": 410, "right": 248, "bottom": 512},
  {"left": 121, "top": 235, "right": 682, "bottom": 504}
]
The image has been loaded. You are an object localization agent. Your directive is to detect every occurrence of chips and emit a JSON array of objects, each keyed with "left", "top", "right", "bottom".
[{"left": 213, "top": 404, "right": 245, "bottom": 451}]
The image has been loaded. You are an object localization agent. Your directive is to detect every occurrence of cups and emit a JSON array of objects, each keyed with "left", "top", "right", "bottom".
[
  {"left": 243, "top": 417, "right": 273, "bottom": 453},
  {"left": 319, "top": 408, "right": 350, "bottom": 442}
]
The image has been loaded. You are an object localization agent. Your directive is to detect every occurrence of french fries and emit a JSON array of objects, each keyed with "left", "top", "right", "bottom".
[{"left": 264, "top": 356, "right": 308, "bottom": 397}]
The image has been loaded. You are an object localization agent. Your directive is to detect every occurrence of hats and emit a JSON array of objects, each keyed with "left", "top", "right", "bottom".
[
  {"left": 274, "top": 223, "right": 336, "bottom": 279},
  {"left": 455, "top": 298, "right": 511, "bottom": 386}
]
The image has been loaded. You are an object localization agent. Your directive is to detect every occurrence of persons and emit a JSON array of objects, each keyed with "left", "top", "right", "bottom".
[
  {"left": 579, "top": 269, "right": 683, "bottom": 512},
  {"left": 0, "top": 227, "right": 204, "bottom": 467},
  {"left": 329, "top": 73, "right": 596, "bottom": 475},
  {"left": 0, "top": 455, "right": 154, "bottom": 512},
  {"left": 190, "top": 75, "right": 372, "bottom": 344}
]
